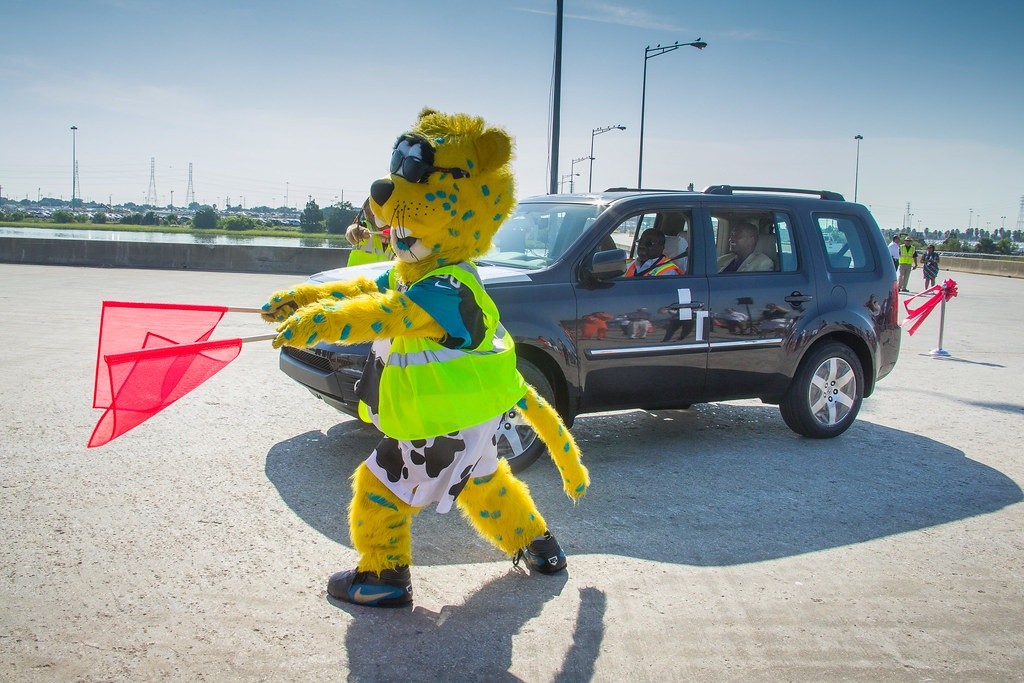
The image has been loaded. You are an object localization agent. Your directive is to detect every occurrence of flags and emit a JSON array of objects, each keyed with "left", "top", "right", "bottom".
[{"left": 87, "top": 301, "right": 242, "bottom": 448}]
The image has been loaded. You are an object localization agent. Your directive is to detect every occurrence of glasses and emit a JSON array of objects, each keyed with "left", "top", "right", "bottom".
[
  {"left": 635, "top": 238, "right": 657, "bottom": 248},
  {"left": 389, "top": 151, "right": 460, "bottom": 184}
]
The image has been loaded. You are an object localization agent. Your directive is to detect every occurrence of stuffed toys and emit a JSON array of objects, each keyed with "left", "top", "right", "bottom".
[{"left": 262, "top": 107, "right": 590, "bottom": 607}]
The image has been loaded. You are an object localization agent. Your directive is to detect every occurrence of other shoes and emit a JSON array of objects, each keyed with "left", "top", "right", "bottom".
[
  {"left": 902, "top": 289, "right": 909, "bottom": 292},
  {"left": 898, "top": 288, "right": 900, "bottom": 292}
]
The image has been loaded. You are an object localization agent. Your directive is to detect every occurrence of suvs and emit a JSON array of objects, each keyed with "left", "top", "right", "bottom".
[{"left": 280, "top": 184, "right": 902, "bottom": 474}]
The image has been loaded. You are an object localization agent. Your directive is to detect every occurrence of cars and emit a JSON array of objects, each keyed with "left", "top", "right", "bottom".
[{"left": 0, "top": 206, "right": 301, "bottom": 225}]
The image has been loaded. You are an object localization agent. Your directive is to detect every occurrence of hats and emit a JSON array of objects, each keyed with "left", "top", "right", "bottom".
[{"left": 904, "top": 236, "right": 912, "bottom": 241}]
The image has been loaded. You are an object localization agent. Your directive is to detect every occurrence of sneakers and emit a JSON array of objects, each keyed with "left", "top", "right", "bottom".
[
  {"left": 512, "top": 531, "right": 568, "bottom": 575},
  {"left": 327, "top": 565, "right": 413, "bottom": 609}
]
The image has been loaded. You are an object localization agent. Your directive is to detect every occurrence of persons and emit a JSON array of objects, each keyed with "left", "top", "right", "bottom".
[
  {"left": 756, "top": 302, "right": 790, "bottom": 337},
  {"left": 346, "top": 197, "right": 398, "bottom": 267},
  {"left": 888, "top": 235, "right": 918, "bottom": 292},
  {"left": 538, "top": 335, "right": 570, "bottom": 366},
  {"left": 920, "top": 245, "right": 940, "bottom": 294},
  {"left": 581, "top": 312, "right": 613, "bottom": 339},
  {"left": 658, "top": 302, "right": 714, "bottom": 341},
  {"left": 722, "top": 307, "right": 749, "bottom": 333},
  {"left": 626, "top": 228, "right": 683, "bottom": 276},
  {"left": 718, "top": 223, "right": 774, "bottom": 272},
  {"left": 616, "top": 306, "right": 651, "bottom": 340},
  {"left": 867, "top": 290, "right": 894, "bottom": 330}
]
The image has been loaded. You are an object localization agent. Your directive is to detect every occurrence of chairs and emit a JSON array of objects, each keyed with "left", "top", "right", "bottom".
[
  {"left": 664, "top": 234, "right": 688, "bottom": 274},
  {"left": 758, "top": 233, "right": 779, "bottom": 271}
]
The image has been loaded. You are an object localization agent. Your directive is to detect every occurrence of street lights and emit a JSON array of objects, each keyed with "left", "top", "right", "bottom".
[
  {"left": 589, "top": 126, "right": 627, "bottom": 193},
  {"left": 71, "top": 125, "right": 78, "bottom": 215},
  {"left": 638, "top": 40, "right": 708, "bottom": 191},
  {"left": 570, "top": 156, "right": 596, "bottom": 194},
  {"left": 909, "top": 214, "right": 914, "bottom": 228},
  {"left": 987, "top": 222, "right": 991, "bottom": 230},
  {"left": 918, "top": 220, "right": 922, "bottom": 231},
  {"left": 1001, "top": 216, "right": 1006, "bottom": 230},
  {"left": 171, "top": 190, "right": 175, "bottom": 213},
  {"left": 853, "top": 135, "right": 863, "bottom": 202},
  {"left": 561, "top": 174, "right": 580, "bottom": 194}
]
